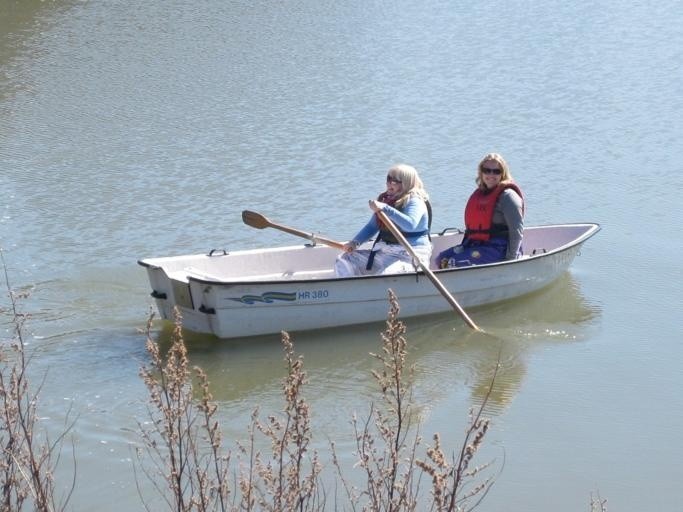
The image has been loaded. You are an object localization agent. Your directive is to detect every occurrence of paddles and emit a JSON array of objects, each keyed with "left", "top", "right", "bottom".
[{"left": 242, "top": 210, "right": 353, "bottom": 252}]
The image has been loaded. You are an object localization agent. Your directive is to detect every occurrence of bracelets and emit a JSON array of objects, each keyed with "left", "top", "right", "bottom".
[{"left": 347, "top": 240, "right": 359, "bottom": 248}]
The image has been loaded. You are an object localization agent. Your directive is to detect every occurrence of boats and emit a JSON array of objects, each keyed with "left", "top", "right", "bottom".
[{"left": 135, "top": 220, "right": 604, "bottom": 341}]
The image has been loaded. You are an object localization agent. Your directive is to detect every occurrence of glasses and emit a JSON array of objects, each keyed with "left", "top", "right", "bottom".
[
  {"left": 386, "top": 175, "right": 401, "bottom": 184},
  {"left": 481, "top": 167, "right": 501, "bottom": 175}
]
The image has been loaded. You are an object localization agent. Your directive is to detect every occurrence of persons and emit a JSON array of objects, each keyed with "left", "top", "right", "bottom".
[
  {"left": 333, "top": 163, "right": 432, "bottom": 275},
  {"left": 434, "top": 151, "right": 524, "bottom": 270},
  {"left": 368, "top": 358, "right": 436, "bottom": 425},
  {"left": 469, "top": 347, "right": 530, "bottom": 422}
]
